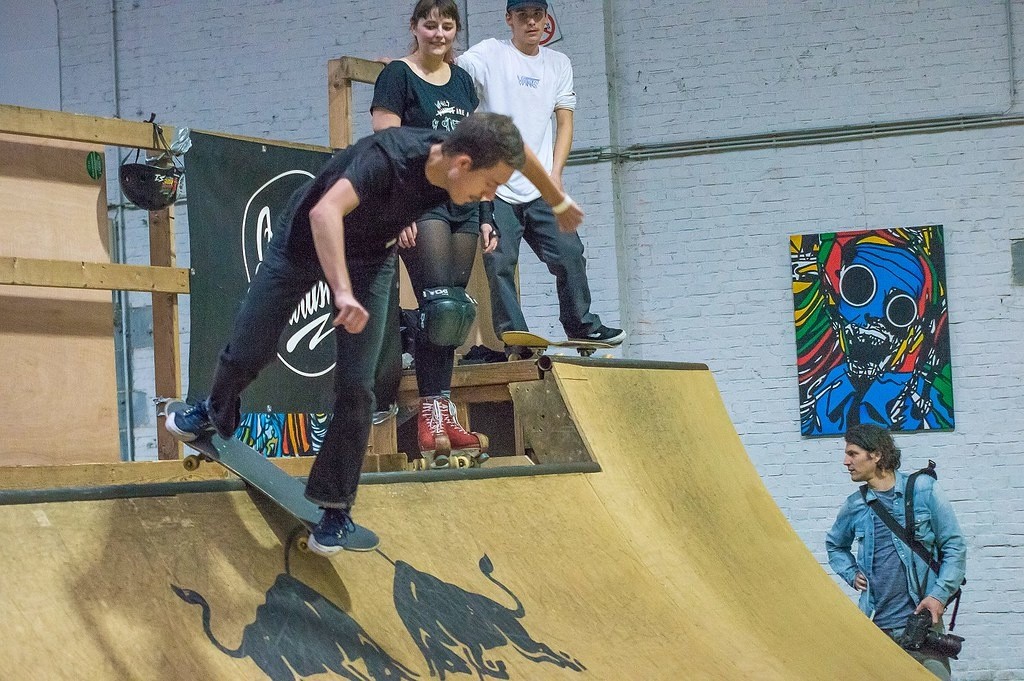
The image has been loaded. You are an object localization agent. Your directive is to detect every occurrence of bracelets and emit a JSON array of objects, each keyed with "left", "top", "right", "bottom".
[{"left": 552, "top": 194, "right": 575, "bottom": 214}]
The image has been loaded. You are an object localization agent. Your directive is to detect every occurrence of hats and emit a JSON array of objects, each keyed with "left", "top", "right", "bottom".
[{"left": 506, "top": 0, "right": 550, "bottom": 11}]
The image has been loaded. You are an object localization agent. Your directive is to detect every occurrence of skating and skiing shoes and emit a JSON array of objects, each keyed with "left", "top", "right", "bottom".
[
  {"left": 439, "top": 399, "right": 489, "bottom": 468},
  {"left": 412, "top": 397, "right": 459, "bottom": 471}
]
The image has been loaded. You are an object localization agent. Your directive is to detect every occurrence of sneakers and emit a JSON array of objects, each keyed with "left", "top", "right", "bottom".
[
  {"left": 307, "top": 505, "right": 354, "bottom": 557},
  {"left": 568, "top": 324, "right": 626, "bottom": 343},
  {"left": 165, "top": 400, "right": 217, "bottom": 441},
  {"left": 505, "top": 345, "right": 540, "bottom": 361}
]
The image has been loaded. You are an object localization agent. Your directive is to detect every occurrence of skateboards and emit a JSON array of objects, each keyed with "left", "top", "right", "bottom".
[
  {"left": 165, "top": 400, "right": 379, "bottom": 552},
  {"left": 501, "top": 331, "right": 623, "bottom": 357}
]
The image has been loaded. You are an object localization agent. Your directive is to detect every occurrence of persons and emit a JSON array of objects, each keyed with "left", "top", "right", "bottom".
[
  {"left": 370, "top": 1, "right": 489, "bottom": 456},
  {"left": 361, "top": 1, "right": 628, "bottom": 361},
  {"left": 166, "top": 111, "right": 587, "bottom": 555},
  {"left": 824, "top": 422, "right": 967, "bottom": 681}
]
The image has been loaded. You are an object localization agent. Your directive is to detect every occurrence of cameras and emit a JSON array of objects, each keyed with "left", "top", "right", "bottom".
[{"left": 900, "top": 610, "right": 968, "bottom": 661}]
